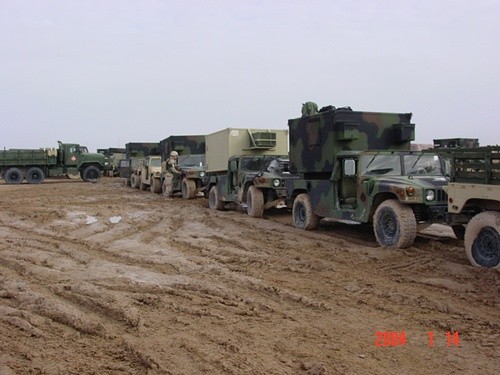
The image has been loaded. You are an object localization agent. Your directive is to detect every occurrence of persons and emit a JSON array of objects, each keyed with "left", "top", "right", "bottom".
[
  {"left": 420, "top": 158, "right": 440, "bottom": 176},
  {"left": 167, "top": 151, "right": 181, "bottom": 184}
]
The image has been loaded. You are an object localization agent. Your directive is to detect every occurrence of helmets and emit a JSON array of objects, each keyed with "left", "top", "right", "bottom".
[{"left": 170, "top": 151, "right": 178, "bottom": 157}]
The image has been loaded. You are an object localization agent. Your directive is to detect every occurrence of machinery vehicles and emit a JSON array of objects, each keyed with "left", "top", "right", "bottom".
[
  {"left": 0, "top": 140, "right": 110, "bottom": 186},
  {"left": 119, "top": 142, "right": 161, "bottom": 194},
  {"left": 160, "top": 134, "right": 205, "bottom": 201},
  {"left": 285, "top": 101, "right": 470, "bottom": 249},
  {"left": 205, "top": 127, "right": 291, "bottom": 217}
]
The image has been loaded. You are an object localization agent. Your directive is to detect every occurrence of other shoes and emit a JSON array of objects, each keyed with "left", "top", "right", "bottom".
[{"left": 163, "top": 196, "right": 173, "bottom": 200}]
